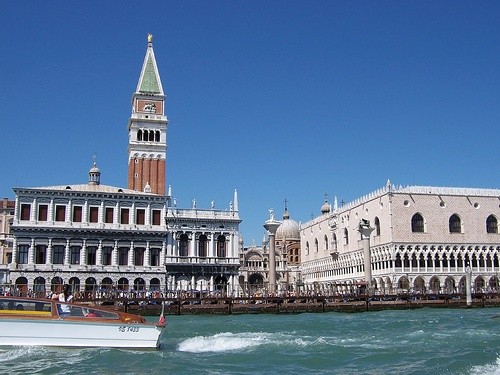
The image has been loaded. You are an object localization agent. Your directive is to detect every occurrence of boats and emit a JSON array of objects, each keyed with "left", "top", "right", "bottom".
[{"left": 0, "top": 294, "right": 168, "bottom": 349}]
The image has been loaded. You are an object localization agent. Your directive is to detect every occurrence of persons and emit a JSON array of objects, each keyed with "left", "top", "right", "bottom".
[
  {"left": 0, "top": 271, "right": 499, "bottom": 302},
  {"left": 49, "top": 284, "right": 81, "bottom": 318}
]
